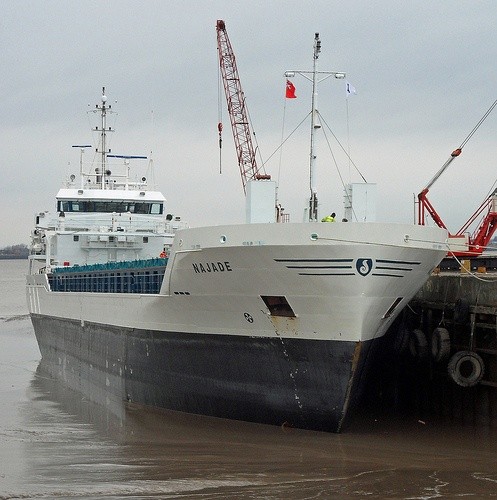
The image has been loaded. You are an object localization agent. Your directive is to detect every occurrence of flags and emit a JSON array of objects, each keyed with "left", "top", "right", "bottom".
[
  {"left": 285, "top": 80, "right": 297, "bottom": 99},
  {"left": 346, "top": 81, "right": 359, "bottom": 98}
]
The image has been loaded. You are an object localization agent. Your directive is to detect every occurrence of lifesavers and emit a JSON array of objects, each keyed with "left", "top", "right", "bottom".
[
  {"left": 432, "top": 328, "right": 450, "bottom": 362},
  {"left": 409, "top": 328, "right": 427, "bottom": 357},
  {"left": 448, "top": 351, "right": 485, "bottom": 387}
]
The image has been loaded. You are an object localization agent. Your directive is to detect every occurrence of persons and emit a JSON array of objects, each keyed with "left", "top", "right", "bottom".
[
  {"left": 160, "top": 248, "right": 167, "bottom": 258},
  {"left": 341, "top": 218, "right": 349, "bottom": 223},
  {"left": 321, "top": 213, "right": 336, "bottom": 223}
]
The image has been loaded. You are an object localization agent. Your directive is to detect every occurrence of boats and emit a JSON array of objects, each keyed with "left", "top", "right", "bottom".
[
  {"left": 216, "top": 18, "right": 497, "bottom": 386},
  {"left": 24, "top": 86, "right": 450, "bottom": 435}
]
